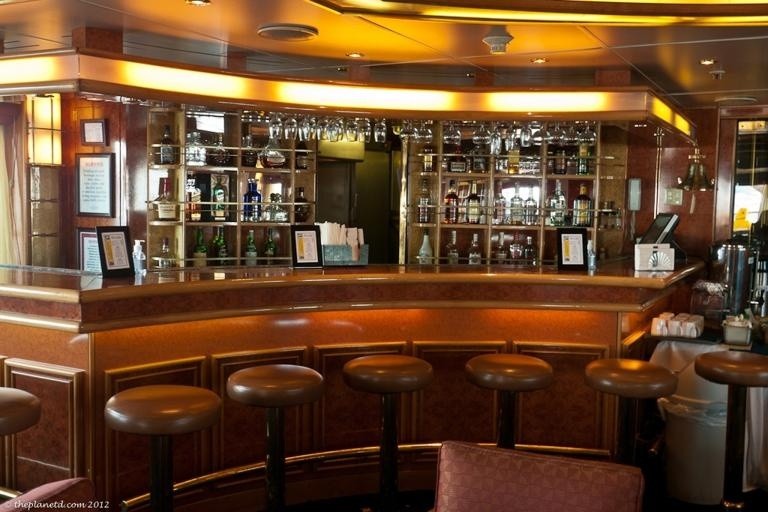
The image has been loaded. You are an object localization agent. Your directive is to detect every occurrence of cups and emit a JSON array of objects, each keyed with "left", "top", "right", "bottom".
[
  {"left": 312, "top": 221, "right": 364, "bottom": 262},
  {"left": 563, "top": 235, "right": 570, "bottom": 261},
  {"left": 297, "top": 233, "right": 305, "bottom": 259},
  {"left": 649, "top": 296, "right": 705, "bottom": 341}
]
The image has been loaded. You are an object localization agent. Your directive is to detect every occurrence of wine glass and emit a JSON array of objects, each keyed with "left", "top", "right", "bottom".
[{"left": 268, "top": 114, "right": 599, "bottom": 155}]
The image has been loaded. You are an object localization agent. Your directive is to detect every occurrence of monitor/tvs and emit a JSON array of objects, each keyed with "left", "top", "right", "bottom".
[{"left": 638, "top": 212, "right": 687, "bottom": 264}]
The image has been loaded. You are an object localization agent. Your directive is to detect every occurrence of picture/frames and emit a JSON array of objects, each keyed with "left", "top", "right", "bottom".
[
  {"left": 73, "top": 151, "right": 117, "bottom": 220},
  {"left": 78, "top": 116, "right": 110, "bottom": 147},
  {"left": 75, "top": 225, "right": 102, "bottom": 274},
  {"left": 95, "top": 222, "right": 134, "bottom": 279},
  {"left": 556, "top": 227, "right": 589, "bottom": 274},
  {"left": 290, "top": 223, "right": 322, "bottom": 270}
]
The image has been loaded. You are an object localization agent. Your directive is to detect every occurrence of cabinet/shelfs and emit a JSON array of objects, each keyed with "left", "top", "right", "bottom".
[
  {"left": 397, "top": 127, "right": 603, "bottom": 265},
  {"left": 145, "top": 107, "right": 320, "bottom": 270}
]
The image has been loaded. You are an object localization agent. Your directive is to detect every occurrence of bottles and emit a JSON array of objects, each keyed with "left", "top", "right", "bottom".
[
  {"left": 494, "top": 232, "right": 508, "bottom": 263},
  {"left": 193, "top": 226, "right": 276, "bottom": 266},
  {"left": 157, "top": 179, "right": 178, "bottom": 220},
  {"left": 615, "top": 208, "right": 623, "bottom": 228},
  {"left": 184, "top": 172, "right": 311, "bottom": 222},
  {"left": 587, "top": 240, "right": 596, "bottom": 271},
  {"left": 159, "top": 123, "right": 310, "bottom": 169},
  {"left": 415, "top": 179, "right": 430, "bottom": 221},
  {"left": 158, "top": 238, "right": 176, "bottom": 266},
  {"left": 422, "top": 145, "right": 591, "bottom": 174},
  {"left": 446, "top": 230, "right": 460, "bottom": 265},
  {"left": 509, "top": 232, "right": 524, "bottom": 264},
  {"left": 418, "top": 229, "right": 434, "bottom": 265},
  {"left": 469, "top": 233, "right": 482, "bottom": 265},
  {"left": 525, "top": 235, "right": 537, "bottom": 266},
  {"left": 442, "top": 179, "right": 593, "bottom": 227}
]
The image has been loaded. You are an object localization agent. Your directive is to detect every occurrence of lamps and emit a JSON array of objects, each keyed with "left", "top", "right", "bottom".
[
  {"left": 255, "top": 19, "right": 320, "bottom": 44},
  {"left": 672, "top": 147, "right": 720, "bottom": 194}
]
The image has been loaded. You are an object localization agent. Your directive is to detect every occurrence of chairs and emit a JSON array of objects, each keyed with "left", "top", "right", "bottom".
[
  {"left": 430, "top": 437, "right": 647, "bottom": 510},
  {"left": 0, "top": 473, "right": 96, "bottom": 512}
]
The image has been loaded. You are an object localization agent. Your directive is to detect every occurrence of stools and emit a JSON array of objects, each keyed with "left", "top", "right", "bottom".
[
  {"left": 341, "top": 353, "right": 435, "bottom": 507},
  {"left": 462, "top": 345, "right": 557, "bottom": 457},
  {"left": 102, "top": 380, "right": 222, "bottom": 508},
  {"left": 691, "top": 346, "right": 768, "bottom": 508},
  {"left": 580, "top": 354, "right": 683, "bottom": 469},
  {"left": 223, "top": 357, "right": 326, "bottom": 511},
  {"left": 0, "top": 382, "right": 43, "bottom": 499}
]
